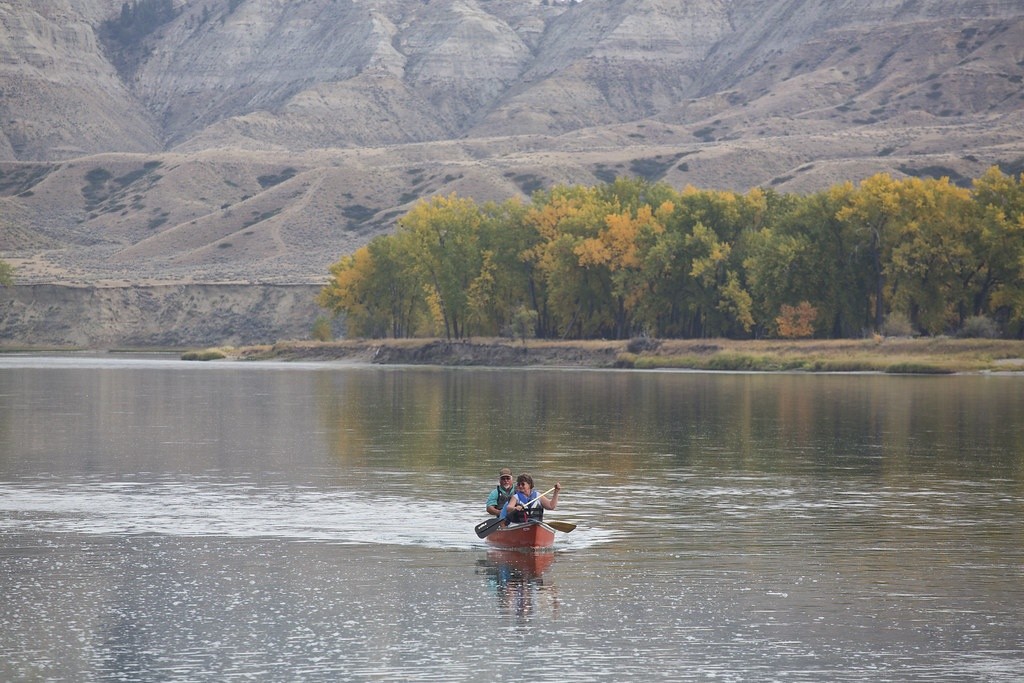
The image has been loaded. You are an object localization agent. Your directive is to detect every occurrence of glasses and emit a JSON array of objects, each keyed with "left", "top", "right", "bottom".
[
  {"left": 500, "top": 478, "right": 511, "bottom": 480},
  {"left": 517, "top": 482, "right": 526, "bottom": 486}
]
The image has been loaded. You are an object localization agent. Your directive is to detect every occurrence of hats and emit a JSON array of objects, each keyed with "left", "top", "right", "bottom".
[{"left": 500, "top": 468, "right": 512, "bottom": 478}]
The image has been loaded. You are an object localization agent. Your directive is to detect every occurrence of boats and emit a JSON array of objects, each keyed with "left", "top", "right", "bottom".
[
  {"left": 485, "top": 547, "right": 556, "bottom": 580},
  {"left": 485, "top": 521, "right": 555, "bottom": 547}
]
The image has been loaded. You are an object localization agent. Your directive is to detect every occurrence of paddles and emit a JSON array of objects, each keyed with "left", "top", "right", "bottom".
[
  {"left": 476, "top": 483, "right": 558, "bottom": 538},
  {"left": 522, "top": 518, "right": 578, "bottom": 531}
]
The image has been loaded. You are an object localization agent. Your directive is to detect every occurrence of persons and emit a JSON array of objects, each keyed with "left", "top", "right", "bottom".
[
  {"left": 506, "top": 473, "right": 561, "bottom": 522},
  {"left": 486, "top": 469, "right": 517, "bottom": 519}
]
